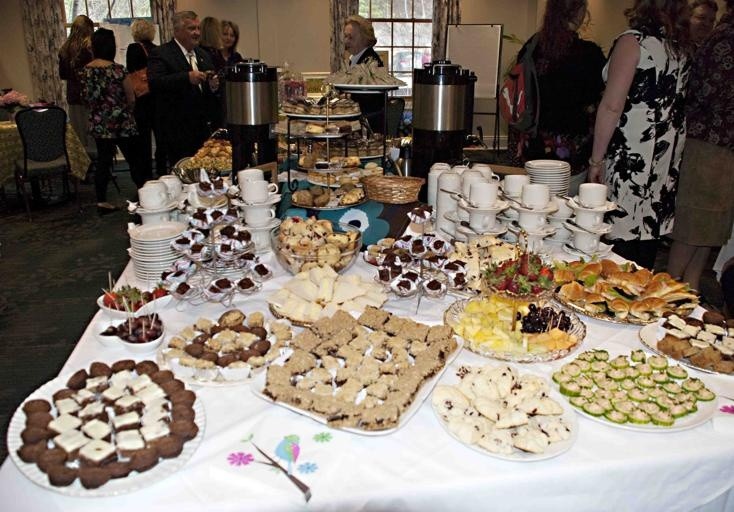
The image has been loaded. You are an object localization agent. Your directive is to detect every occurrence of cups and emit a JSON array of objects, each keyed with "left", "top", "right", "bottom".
[
  {"left": 427, "top": 158, "right": 614, "bottom": 260},
  {"left": 92, "top": 322, "right": 165, "bottom": 353},
  {"left": 134, "top": 170, "right": 281, "bottom": 254}
]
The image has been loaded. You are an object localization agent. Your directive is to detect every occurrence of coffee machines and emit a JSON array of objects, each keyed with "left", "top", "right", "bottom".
[
  {"left": 407, "top": 61, "right": 476, "bottom": 201},
  {"left": 223, "top": 58, "right": 277, "bottom": 184}
]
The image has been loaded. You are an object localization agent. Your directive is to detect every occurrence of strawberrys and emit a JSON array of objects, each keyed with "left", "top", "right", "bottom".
[
  {"left": 479, "top": 252, "right": 553, "bottom": 297},
  {"left": 103, "top": 282, "right": 167, "bottom": 312}
]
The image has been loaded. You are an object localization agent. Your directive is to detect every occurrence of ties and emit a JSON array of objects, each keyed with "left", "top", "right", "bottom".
[{"left": 189, "top": 52, "right": 203, "bottom": 94}]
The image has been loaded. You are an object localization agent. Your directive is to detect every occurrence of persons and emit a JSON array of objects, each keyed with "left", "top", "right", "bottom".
[
  {"left": 219, "top": 19, "right": 242, "bottom": 66},
  {"left": 124, "top": 17, "right": 169, "bottom": 177},
  {"left": 78, "top": 28, "right": 153, "bottom": 216},
  {"left": 57, "top": 14, "right": 98, "bottom": 183},
  {"left": 342, "top": 15, "right": 390, "bottom": 140},
  {"left": 146, "top": 10, "right": 225, "bottom": 171},
  {"left": 198, "top": 16, "right": 233, "bottom": 132}
]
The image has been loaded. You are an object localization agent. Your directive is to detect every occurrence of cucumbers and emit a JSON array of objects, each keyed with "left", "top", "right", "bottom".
[{"left": 552, "top": 348, "right": 716, "bottom": 426}]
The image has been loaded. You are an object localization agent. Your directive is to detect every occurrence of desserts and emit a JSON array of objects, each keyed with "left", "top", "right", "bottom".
[
  {"left": 432, "top": 363, "right": 572, "bottom": 454},
  {"left": 291, "top": 182, "right": 365, "bottom": 205},
  {"left": 160, "top": 169, "right": 272, "bottom": 301},
  {"left": 163, "top": 309, "right": 295, "bottom": 381},
  {"left": 283, "top": 93, "right": 360, "bottom": 114},
  {"left": 655, "top": 310, "right": 732, "bottom": 374},
  {"left": 264, "top": 305, "right": 459, "bottom": 432},
  {"left": 268, "top": 264, "right": 389, "bottom": 326},
  {"left": 380, "top": 233, "right": 467, "bottom": 295},
  {"left": 297, "top": 120, "right": 384, "bottom": 184},
  {"left": 17, "top": 360, "right": 199, "bottom": 489}
]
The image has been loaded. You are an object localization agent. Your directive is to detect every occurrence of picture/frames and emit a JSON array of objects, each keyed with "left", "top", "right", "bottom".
[{"left": 299, "top": 71, "right": 330, "bottom": 97}]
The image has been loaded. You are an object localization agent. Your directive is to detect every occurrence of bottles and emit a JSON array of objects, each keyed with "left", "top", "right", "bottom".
[{"left": 401, "top": 143, "right": 411, "bottom": 178}]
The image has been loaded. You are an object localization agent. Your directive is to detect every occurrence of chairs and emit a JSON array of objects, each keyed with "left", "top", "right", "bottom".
[{"left": 11, "top": 105, "right": 84, "bottom": 225}]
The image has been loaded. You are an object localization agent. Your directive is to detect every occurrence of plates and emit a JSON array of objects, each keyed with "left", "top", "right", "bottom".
[
  {"left": 332, "top": 82, "right": 398, "bottom": 95},
  {"left": 554, "top": 377, "right": 718, "bottom": 432},
  {"left": 6, "top": 370, "right": 204, "bottom": 497},
  {"left": 250, "top": 332, "right": 463, "bottom": 435},
  {"left": 482, "top": 275, "right": 556, "bottom": 300},
  {"left": 556, "top": 262, "right": 699, "bottom": 325},
  {"left": 269, "top": 278, "right": 388, "bottom": 326},
  {"left": 125, "top": 219, "right": 252, "bottom": 305},
  {"left": 154, "top": 317, "right": 290, "bottom": 385},
  {"left": 430, "top": 383, "right": 579, "bottom": 463},
  {"left": 281, "top": 86, "right": 399, "bottom": 213},
  {"left": 640, "top": 323, "right": 733, "bottom": 377},
  {"left": 444, "top": 295, "right": 587, "bottom": 361},
  {"left": 97, "top": 293, "right": 173, "bottom": 316}
]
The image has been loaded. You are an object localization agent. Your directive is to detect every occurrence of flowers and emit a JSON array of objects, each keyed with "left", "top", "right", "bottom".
[{"left": 0, "top": 87, "right": 33, "bottom": 113}]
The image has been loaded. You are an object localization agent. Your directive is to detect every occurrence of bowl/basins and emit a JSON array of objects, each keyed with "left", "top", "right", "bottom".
[{"left": 269, "top": 221, "right": 362, "bottom": 277}]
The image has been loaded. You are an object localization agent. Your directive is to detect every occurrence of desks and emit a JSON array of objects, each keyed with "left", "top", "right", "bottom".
[{"left": 1, "top": 120, "right": 92, "bottom": 214}]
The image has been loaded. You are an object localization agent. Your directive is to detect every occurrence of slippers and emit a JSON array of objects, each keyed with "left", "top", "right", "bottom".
[{"left": 96, "top": 206, "right": 120, "bottom": 213}]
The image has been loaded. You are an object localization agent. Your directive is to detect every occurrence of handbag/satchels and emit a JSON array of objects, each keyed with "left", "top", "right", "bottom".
[{"left": 127, "top": 41, "right": 151, "bottom": 98}]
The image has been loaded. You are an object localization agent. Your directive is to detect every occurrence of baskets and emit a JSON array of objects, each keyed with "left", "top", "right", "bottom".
[{"left": 359, "top": 175, "right": 426, "bottom": 205}]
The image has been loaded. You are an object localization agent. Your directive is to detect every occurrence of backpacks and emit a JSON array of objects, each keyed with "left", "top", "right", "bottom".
[{"left": 499, "top": 32, "right": 540, "bottom": 158}]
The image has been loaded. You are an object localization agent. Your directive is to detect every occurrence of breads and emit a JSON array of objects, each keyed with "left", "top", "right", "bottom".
[
  {"left": 552, "top": 256, "right": 701, "bottom": 319},
  {"left": 271, "top": 216, "right": 362, "bottom": 276}
]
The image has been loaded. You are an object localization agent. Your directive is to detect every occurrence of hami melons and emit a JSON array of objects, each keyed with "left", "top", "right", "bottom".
[{"left": 458, "top": 292, "right": 575, "bottom": 352}]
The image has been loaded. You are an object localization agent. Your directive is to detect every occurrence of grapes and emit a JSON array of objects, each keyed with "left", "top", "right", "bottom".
[{"left": 100, "top": 312, "right": 162, "bottom": 343}]
